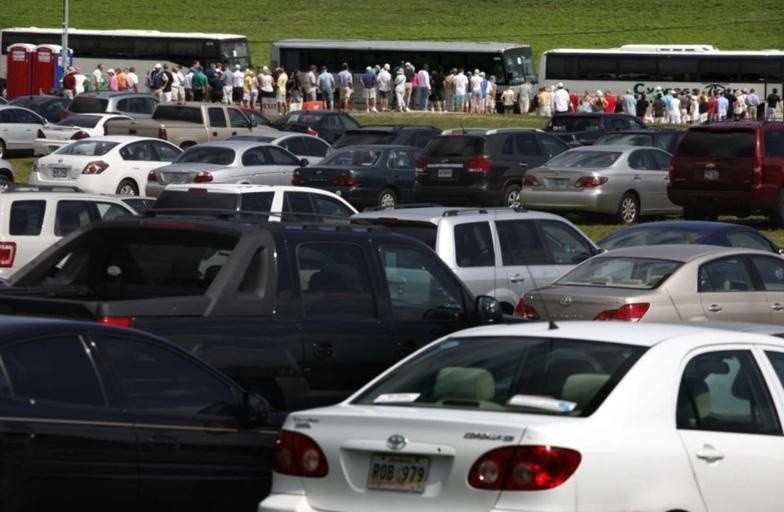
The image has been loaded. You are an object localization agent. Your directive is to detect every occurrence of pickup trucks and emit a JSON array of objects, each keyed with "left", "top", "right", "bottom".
[{"left": 1, "top": 209, "right": 532, "bottom": 411}]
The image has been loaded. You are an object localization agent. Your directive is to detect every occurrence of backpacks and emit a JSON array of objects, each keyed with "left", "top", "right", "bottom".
[{"left": 152, "top": 72, "right": 164, "bottom": 88}]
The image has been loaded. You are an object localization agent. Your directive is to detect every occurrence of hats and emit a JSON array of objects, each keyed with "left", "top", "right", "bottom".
[
  {"left": 154, "top": 64, "right": 162, "bottom": 69},
  {"left": 383, "top": 63, "right": 415, "bottom": 75}
]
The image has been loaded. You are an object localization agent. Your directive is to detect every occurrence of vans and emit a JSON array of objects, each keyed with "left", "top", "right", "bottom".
[{"left": 150, "top": 182, "right": 357, "bottom": 230}]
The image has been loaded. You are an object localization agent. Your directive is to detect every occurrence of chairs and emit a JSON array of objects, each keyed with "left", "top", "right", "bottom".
[
  {"left": 678, "top": 377, "right": 714, "bottom": 430},
  {"left": 432, "top": 365, "right": 495, "bottom": 404},
  {"left": 562, "top": 372, "right": 611, "bottom": 409},
  {"left": 545, "top": 359, "right": 595, "bottom": 400}
]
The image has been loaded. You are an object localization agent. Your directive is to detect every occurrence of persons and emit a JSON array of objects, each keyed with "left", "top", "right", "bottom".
[
  {"left": 500, "top": 80, "right": 784, "bottom": 125},
  {"left": 61, "top": 63, "right": 138, "bottom": 101},
  {"left": 360, "top": 60, "right": 498, "bottom": 116},
  {"left": 144, "top": 60, "right": 354, "bottom": 117}
]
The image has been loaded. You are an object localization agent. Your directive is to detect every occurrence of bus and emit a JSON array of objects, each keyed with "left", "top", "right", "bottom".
[
  {"left": 0, "top": 25, "right": 249, "bottom": 94},
  {"left": 271, "top": 40, "right": 536, "bottom": 98},
  {"left": 539, "top": 44, "right": 783, "bottom": 96}
]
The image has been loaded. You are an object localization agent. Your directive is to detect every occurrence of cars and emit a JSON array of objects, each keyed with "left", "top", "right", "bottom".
[
  {"left": 2, "top": 316, "right": 286, "bottom": 508},
  {"left": 254, "top": 322, "right": 784, "bottom": 510},
  {"left": 1, "top": 189, "right": 139, "bottom": 284},
  {"left": 355, "top": 204, "right": 604, "bottom": 309},
  {"left": 508, "top": 244, "right": 783, "bottom": 323}
]
